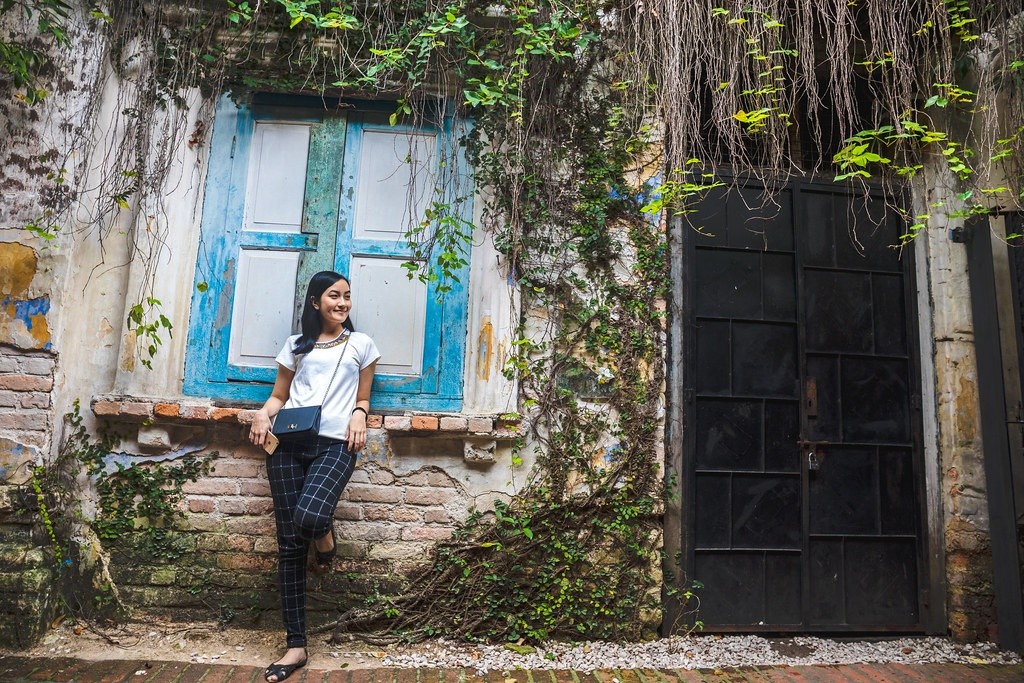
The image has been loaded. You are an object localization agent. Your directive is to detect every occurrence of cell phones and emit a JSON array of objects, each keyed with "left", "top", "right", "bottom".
[{"left": 264, "top": 429, "right": 280, "bottom": 455}]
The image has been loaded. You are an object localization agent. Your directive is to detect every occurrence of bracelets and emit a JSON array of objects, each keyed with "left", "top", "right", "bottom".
[{"left": 352, "top": 407, "right": 368, "bottom": 422}]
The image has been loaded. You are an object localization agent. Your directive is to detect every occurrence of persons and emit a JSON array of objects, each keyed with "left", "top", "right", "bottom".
[{"left": 247, "top": 270, "right": 382, "bottom": 683}]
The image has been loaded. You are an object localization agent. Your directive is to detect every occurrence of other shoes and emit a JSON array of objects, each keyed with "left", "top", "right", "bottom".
[
  {"left": 315, "top": 525, "right": 336, "bottom": 565},
  {"left": 266, "top": 647, "right": 309, "bottom": 682}
]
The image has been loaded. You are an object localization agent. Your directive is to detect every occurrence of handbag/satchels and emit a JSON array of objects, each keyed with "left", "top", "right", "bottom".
[{"left": 271, "top": 405, "right": 321, "bottom": 440}]
